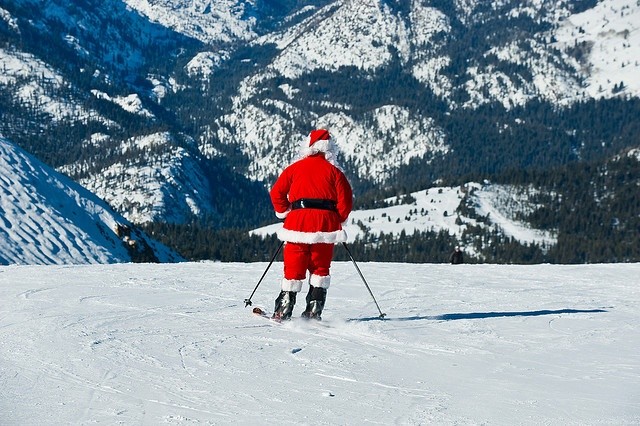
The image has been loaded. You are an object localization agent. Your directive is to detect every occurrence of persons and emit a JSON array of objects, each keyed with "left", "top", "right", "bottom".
[{"left": 269, "top": 127, "right": 354, "bottom": 320}]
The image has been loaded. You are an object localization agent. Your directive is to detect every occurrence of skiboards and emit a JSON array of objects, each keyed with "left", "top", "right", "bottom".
[{"left": 253, "top": 307, "right": 334, "bottom": 330}]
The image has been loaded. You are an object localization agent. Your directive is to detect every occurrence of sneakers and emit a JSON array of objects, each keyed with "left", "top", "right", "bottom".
[
  {"left": 301, "top": 283, "right": 327, "bottom": 320},
  {"left": 271, "top": 289, "right": 297, "bottom": 320}
]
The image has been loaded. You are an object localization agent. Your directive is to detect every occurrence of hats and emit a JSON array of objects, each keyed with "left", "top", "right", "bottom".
[{"left": 306, "top": 129, "right": 332, "bottom": 152}]
